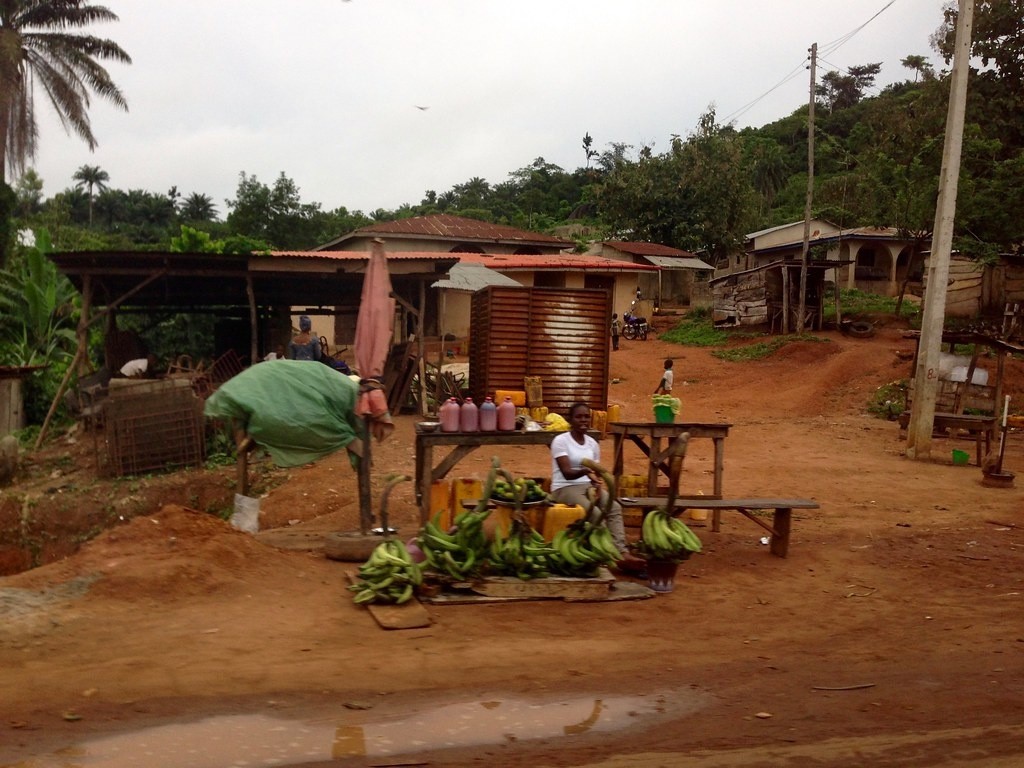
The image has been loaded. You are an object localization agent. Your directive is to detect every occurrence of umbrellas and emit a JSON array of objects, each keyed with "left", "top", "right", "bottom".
[{"left": 352, "top": 238, "right": 395, "bottom": 519}]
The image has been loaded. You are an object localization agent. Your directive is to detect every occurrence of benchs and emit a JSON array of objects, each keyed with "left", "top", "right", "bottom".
[{"left": 617, "top": 496, "right": 822, "bottom": 558}]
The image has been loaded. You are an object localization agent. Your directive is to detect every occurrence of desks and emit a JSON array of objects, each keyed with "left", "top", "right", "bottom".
[
  {"left": 608, "top": 421, "right": 735, "bottom": 533},
  {"left": 898, "top": 410, "right": 997, "bottom": 467},
  {"left": 415, "top": 428, "right": 604, "bottom": 528}
]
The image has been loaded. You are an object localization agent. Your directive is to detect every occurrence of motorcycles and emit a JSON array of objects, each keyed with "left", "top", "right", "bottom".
[{"left": 621, "top": 301, "right": 650, "bottom": 341}]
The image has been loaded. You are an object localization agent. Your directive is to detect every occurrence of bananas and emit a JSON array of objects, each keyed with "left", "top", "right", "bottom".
[{"left": 347, "top": 477, "right": 704, "bottom": 606}]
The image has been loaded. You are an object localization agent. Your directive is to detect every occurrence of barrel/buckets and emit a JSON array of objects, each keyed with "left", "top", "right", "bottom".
[{"left": 651, "top": 397, "right": 678, "bottom": 423}]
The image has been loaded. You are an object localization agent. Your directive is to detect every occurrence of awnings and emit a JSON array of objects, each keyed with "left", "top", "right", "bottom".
[{"left": 644, "top": 255, "right": 716, "bottom": 272}]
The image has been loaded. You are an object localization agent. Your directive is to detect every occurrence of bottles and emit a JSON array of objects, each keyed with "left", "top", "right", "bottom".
[{"left": 439, "top": 396, "right": 516, "bottom": 431}]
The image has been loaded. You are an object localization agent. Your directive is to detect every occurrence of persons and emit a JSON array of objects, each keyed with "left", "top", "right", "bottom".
[
  {"left": 290, "top": 316, "right": 323, "bottom": 361},
  {"left": 551, "top": 404, "right": 648, "bottom": 571},
  {"left": 636, "top": 286, "right": 642, "bottom": 299},
  {"left": 654, "top": 359, "right": 674, "bottom": 396},
  {"left": 609, "top": 313, "right": 622, "bottom": 351},
  {"left": 116, "top": 352, "right": 158, "bottom": 378}
]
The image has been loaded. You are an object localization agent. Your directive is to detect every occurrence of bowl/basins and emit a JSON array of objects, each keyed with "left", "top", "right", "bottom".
[{"left": 418, "top": 421, "right": 439, "bottom": 432}]
